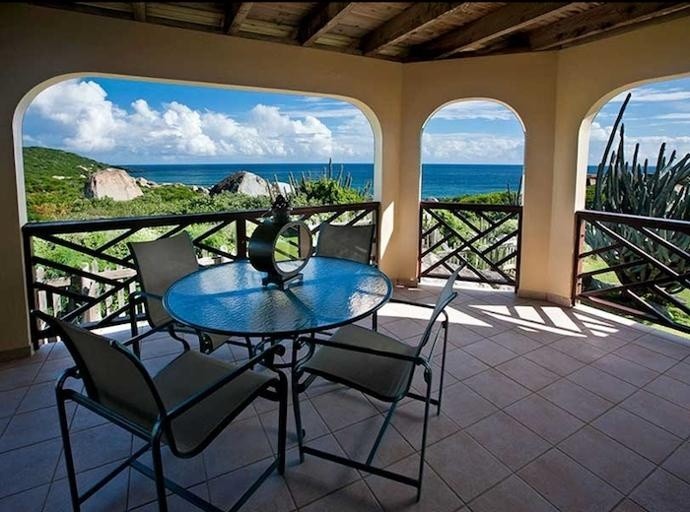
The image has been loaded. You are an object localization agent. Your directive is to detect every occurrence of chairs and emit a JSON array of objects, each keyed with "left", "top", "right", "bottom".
[
  {"left": 315, "top": 220, "right": 379, "bottom": 334},
  {"left": 31, "top": 308, "right": 288, "bottom": 511},
  {"left": 291, "top": 259, "right": 469, "bottom": 504},
  {"left": 126, "top": 230, "right": 256, "bottom": 371}
]
